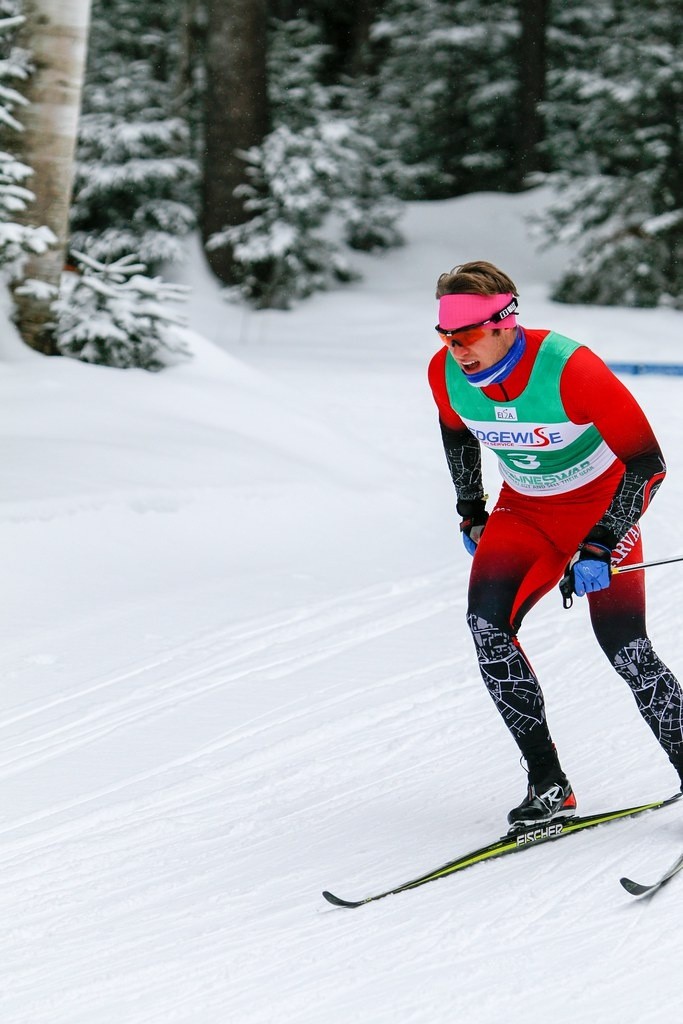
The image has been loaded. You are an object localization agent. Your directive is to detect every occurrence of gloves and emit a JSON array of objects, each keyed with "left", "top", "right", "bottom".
[
  {"left": 564, "top": 525, "right": 619, "bottom": 598},
  {"left": 456, "top": 499, "right": 488, "bottom": 558}
]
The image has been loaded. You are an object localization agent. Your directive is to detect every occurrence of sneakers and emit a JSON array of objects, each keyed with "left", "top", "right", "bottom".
[{"left": 506, "top": 778, "right": 578, "bottom": 826}]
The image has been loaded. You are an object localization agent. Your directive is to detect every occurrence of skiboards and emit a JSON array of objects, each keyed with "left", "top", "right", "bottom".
[{"left": 318, "top": 785, "right": 683, "bottom": 912}]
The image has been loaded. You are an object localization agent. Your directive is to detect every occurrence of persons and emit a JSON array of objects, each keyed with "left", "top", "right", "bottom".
[{"left": 428, "top": 262, "right": 683, "bottom": 826}]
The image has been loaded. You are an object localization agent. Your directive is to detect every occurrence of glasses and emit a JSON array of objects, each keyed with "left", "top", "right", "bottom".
[{"left": 435, "top": 299, "right": 519, "bottom": 349}]
